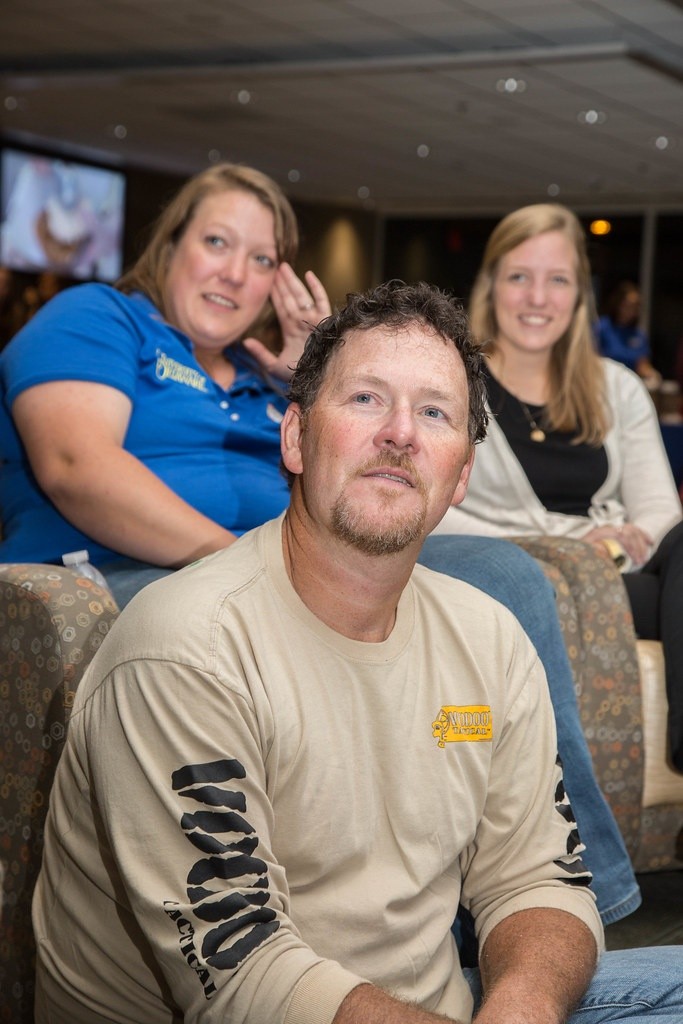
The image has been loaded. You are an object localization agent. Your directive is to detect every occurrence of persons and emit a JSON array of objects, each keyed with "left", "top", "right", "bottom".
[
  {"left": 0, "top": 164, "right": 639, "bottom": 929},
  {"left": 427, "top": 203, "right": 683, "bottom": 803},
  {"left": 31, "top": 282, "right": 683, "bottom": 1024}
]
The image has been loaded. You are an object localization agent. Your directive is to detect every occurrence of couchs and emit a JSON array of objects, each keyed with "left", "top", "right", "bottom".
[{"left": 0, "top": 537, "right": 683, "bottom": 1024}]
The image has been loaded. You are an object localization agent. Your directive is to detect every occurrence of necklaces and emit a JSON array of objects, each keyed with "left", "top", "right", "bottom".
[{"left": 514, "top": 394, "right": 552, "bottom": 443}]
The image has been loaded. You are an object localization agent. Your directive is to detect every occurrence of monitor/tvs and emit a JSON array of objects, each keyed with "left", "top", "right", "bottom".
[{"left": 0, "top": 140, "right": 131, "bottom": 285}]
